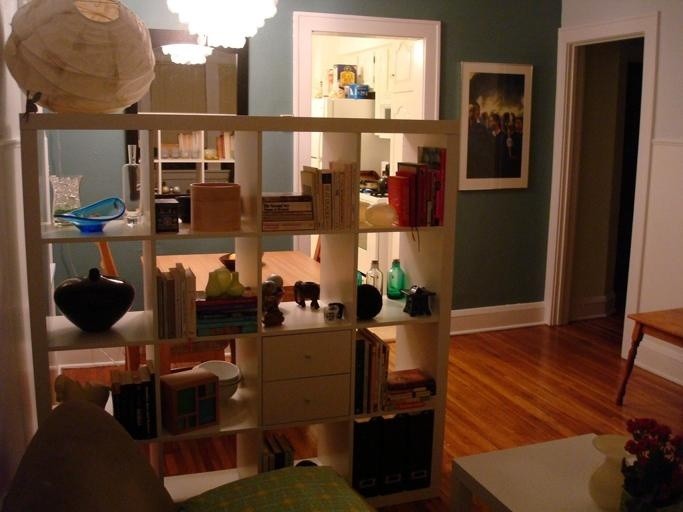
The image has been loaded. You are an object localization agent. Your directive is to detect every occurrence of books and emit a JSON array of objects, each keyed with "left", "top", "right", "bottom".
[
  {"left": 216, "top": 131, "right": 235, "bottom": 160},
  {"left": 263, "top": 431, "right": 296, "bottom": 471},
  {"left": 355, "top": 328, "right": 437, "bottom": 415},
  {"left": 110, "top": 360, "right": 157, "bottom": 441},
  {"left": 387, "top": 146, "right": 446, "bottom": 227},
  {"left": 261, "top": 159, "right": 357, "bottom": 232},
  {"left": 157, "top": 263, "right": 258, "bottom": 339}
]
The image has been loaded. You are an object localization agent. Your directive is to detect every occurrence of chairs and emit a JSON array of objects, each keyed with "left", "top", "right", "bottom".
[{"left": 1, "top": 399, "right": 377, "bottom": 512}]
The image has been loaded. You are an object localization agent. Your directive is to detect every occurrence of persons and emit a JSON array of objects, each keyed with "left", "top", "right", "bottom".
[{"left": 468, "top": 99, "right": 522, "bottom": 178}]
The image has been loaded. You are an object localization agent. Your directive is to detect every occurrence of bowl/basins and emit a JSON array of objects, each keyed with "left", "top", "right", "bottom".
[{"left": 193, "top": 359, "right": 242, "bottom": 401}]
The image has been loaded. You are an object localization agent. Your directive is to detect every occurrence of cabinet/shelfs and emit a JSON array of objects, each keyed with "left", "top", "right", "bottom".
[{"left": 16, "top": 111, "right": 463, "bottom": 507}]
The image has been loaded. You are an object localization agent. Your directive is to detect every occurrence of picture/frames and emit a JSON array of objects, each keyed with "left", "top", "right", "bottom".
[{"left": 458, "top": 60, "right": 534, "bottom": 192}]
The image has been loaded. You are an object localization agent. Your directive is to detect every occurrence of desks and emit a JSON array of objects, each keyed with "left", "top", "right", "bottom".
[{"left": 615, "top": 306, "right": 683, "bottom": 405}]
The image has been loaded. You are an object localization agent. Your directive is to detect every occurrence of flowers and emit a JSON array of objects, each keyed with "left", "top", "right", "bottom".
[{"left": 618, "top": 412, "right": 683, "bottom": 507}]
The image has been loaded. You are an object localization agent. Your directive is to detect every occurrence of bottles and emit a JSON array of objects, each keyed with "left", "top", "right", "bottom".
[
  {"left": 367, "top": 260, "right": 384, "bottom": 297},
  {"left": 121, "top": 144, "right": 140, "bottom": 209},
  {"left": 386, "top": 259, "right": 404, "bottom": 299}
]
